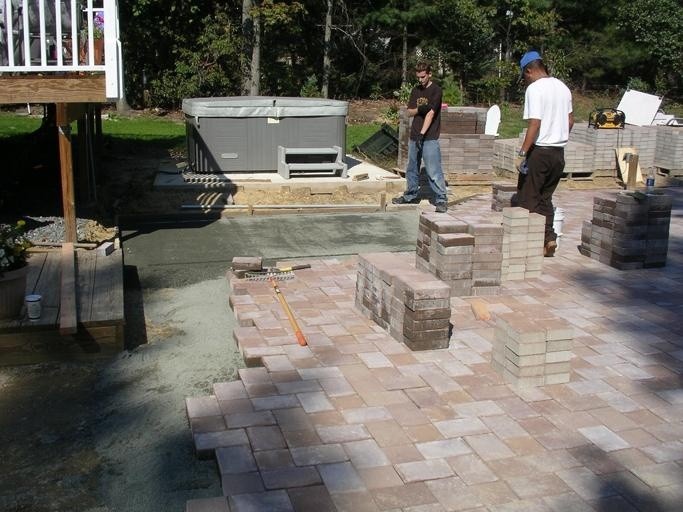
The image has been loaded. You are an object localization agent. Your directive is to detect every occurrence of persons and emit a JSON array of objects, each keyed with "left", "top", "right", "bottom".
[
  {"left": 511, "top": 51, "right": 576, "bottom": 258},
  {"left": 390, "top": 61, "right": 449, "bottom": 214}
]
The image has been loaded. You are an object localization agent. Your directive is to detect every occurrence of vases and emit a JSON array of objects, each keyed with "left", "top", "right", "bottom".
[
  {"left": 0, "top": 262, "right": 31, "bottom": 318},
  {"left": 85, "top": 37, "right": 103, "bottom": 65}
]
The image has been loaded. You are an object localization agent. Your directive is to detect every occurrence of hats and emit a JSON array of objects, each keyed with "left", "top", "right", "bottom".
[{"left": 516, "top": 51, "right": 543, "bottom": 85}]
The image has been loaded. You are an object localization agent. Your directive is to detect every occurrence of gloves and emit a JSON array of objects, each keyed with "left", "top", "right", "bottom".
[
  {"left": 416, "top": 133, "right": 424, "bottom": 151},
  {"left": 515, "top": 156, "right": 528, "bottom": 175},
  {"left": 418, "top": 104, "right": 432, "bottom": 116}
]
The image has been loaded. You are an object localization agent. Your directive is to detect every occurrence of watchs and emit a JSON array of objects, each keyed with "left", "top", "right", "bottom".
[{"left": 519, "top": 150, "right": 526, "bottom": 157}]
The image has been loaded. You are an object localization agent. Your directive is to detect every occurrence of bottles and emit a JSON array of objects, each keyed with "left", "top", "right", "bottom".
[
  {"left": 646, "top": 169, "right": 655, "bottom": 194},
  {"left": 26, "top": 294, "right": 42, "bottom": 322}
]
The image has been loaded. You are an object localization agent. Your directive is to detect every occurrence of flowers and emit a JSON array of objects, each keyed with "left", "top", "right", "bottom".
[
  {"left": 80, "top": 14, "right": 104, "bottom": 46},
  {"left": 0, "top": 220, "right": 35, "bottom": 270}
]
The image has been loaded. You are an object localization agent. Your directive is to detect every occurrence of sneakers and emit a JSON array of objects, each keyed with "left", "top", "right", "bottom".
[
  {"left": 435, "top": 203, "right": 447, "bottom": 213},
  {"left": 544, "top": 241, "right": 557, "bottom": 257},
  {"left": 392, "top": 196, "right": 422, "bottom": 204}
]
